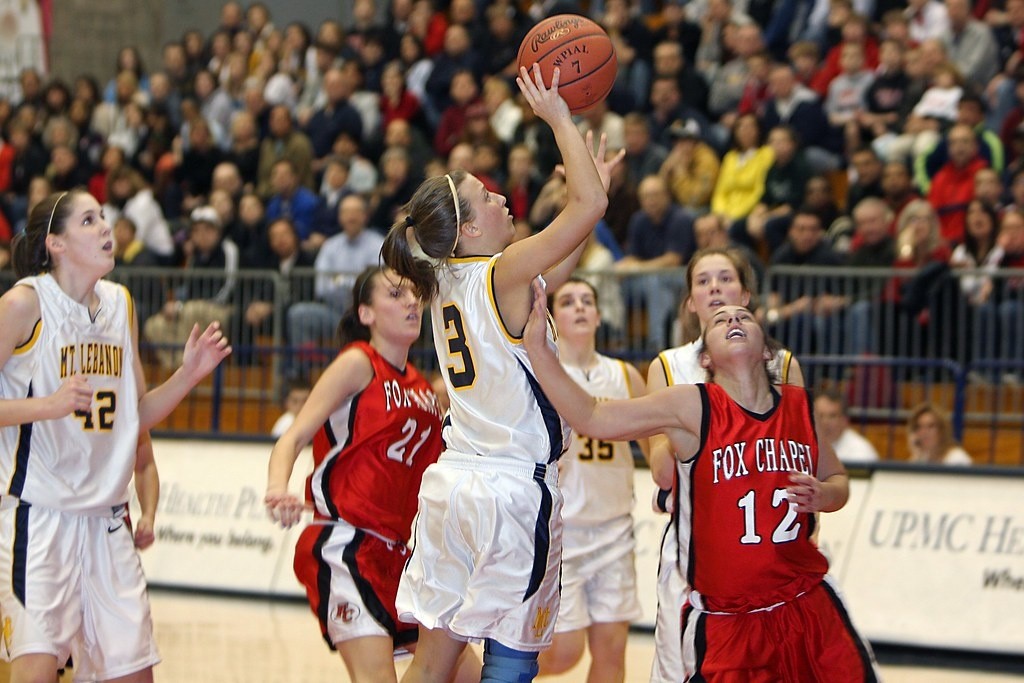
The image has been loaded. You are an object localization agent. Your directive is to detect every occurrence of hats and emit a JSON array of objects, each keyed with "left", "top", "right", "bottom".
[
  {"left": 190, "top": 206, "right": 224, "bottom": 228},
  {"left": 913, "top": 86, "right": 964, "bottom": 124}
]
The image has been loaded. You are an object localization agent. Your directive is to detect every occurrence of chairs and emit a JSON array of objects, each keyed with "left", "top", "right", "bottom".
[{"left": 140, "top": 351, "right": 1023, "bottom": 472}]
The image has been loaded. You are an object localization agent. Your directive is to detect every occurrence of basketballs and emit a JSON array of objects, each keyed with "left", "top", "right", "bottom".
[{"left": 515, "top": 14, "right": 619, "bottom": 115}]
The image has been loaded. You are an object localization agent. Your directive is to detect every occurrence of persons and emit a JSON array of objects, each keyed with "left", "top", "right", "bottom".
[
  {"left": 0, "top": 0, "right": 1023, "bottom": 380},
  {"left": 811, "top": 382, "right": 879, "bottom": 460},
  {"left": 270, "top": 380, "right": 315, "bottom": 439},
  {"left": 263, "top": 262, "right": 444, "bottom": 683},
  {"left": 523, "top": 275, "right": 881, "bottom": 683},
  {"left": 0, "top": 429, "right": 160, "bottom": 683},
  {"left": 906, "top": 404, "right": 973, "bottom": 465},
  {"left": 381, "top": 62, "right": 623, "bottom": 683},
  {"left": 0, "top": 188, "right": 232, "bottom": 683},
  {"left": 650, "top": 242, "right": 805, "bottom": 683},
  {"left": 549, "top": 278, "right": 647, "bottom": 683}
]
